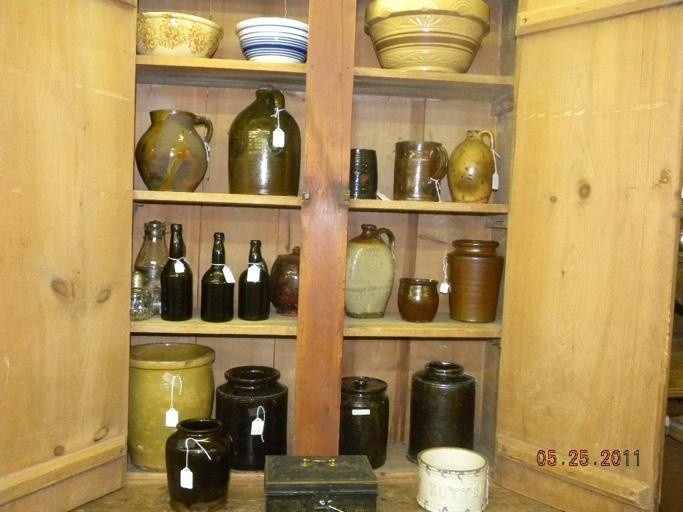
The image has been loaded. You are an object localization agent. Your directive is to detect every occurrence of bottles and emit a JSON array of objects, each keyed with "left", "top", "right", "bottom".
[
  {"left": 215, "top": 365, "right": 288, "bottom": 473},
  {"left": 200, "top": 231, "right": 235, "bottom": 323},
  {"left": 405, "top": 360, "right": 476, "bottom": 466},
  {"left": 134, "top": 220, "right": 168, "bottom": 316},
  {"left": 238, "top": 239, "right": 270, "bottom": 321},
  {"left": 339, "top": 375, "right": 389, "bottom": 470},
  {"left": 159, "top": 223, "right": 193, "bottom": 321}
]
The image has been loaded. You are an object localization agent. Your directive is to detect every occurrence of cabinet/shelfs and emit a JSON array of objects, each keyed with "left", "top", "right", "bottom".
[{"left": 1, "top": 0, "right": 683, "bottom": 512}]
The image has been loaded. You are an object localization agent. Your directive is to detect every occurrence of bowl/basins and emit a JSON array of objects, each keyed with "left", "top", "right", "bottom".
[
  {"left": 137, "top": 11, "right": 224, "bottom": 58},
  {"left": 235, "top": 17, "right": 309, "bottom": 65},
  {"left": 363, "top": 0, "right": 491, "bottom": 73}
]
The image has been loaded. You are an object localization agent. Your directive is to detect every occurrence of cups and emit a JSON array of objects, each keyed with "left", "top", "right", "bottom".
[
  {"left": 394, "top": 142, "right": 449, "bottom": 202},
  {"left": 417, "top": 446, "right": 488, "bottom": 512},
  {"left": 351, "top": 148, "right": 378, "bottom": 199}
]
